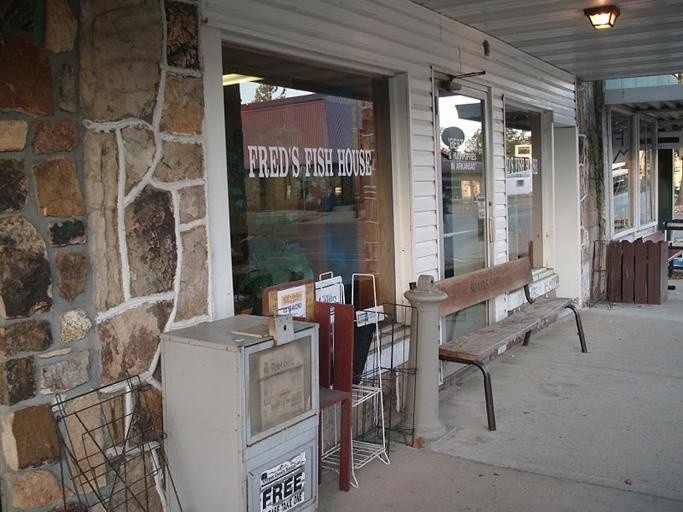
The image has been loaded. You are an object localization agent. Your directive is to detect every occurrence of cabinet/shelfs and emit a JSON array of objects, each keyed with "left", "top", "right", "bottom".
[
  {"left": 356, "top": 301, "right": 419, "bottom": 463},
  {"left": 319, "top": 271, "right": 390, "bottom": 488},
  {"left": 262, "top": 280, "right": 355, "bottom": 491}
]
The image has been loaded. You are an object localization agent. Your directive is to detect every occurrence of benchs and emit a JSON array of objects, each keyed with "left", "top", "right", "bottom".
[
  {"left": 409, "top": 252, "right": 588, "bottom": 430},
  {"left": 642, "top": 232, "right": 683, "bottom": 290}
]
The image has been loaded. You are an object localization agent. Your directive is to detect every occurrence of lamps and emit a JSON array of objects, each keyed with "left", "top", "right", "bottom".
[{"left": 583, "top": 5, "right": 620, "bottom": 31}]
